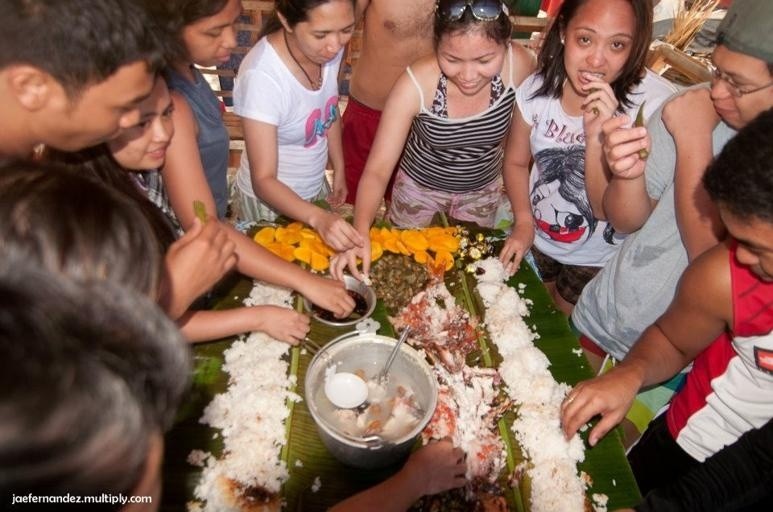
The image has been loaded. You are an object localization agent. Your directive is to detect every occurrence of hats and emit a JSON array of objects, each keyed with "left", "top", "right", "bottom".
[{"left": 716, "top": 0, "right": 773, "bottom": 64}]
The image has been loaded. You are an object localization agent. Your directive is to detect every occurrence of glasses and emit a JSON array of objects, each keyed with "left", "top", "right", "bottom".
[
  {"left": 439, "top": 0, "right": 503, "bottom": 23},
  {"left": 710, "top": 68, "right": 772, "bottom": 96}
]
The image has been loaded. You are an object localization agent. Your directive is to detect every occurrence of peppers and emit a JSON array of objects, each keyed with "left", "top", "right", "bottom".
[
  {"left": 634, "top": 100, "right": 650, "bottom": 161},
  {"left": 588, "top": 88, "right": 598, "bottom": 115}
]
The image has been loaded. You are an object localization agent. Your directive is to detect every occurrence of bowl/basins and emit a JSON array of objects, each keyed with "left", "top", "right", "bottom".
[{"left": 302, "top": 275, "right": 376, "bottom": 328}]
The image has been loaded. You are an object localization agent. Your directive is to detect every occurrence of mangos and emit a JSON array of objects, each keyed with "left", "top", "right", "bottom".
[{"left": 253, "top": 222, "right": 460, "bottom": 272}]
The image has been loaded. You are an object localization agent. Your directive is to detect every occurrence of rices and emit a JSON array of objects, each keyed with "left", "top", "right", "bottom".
[
  {"left": 473, "top": 255, "right": 619, "bottom": 512},
  {"left": 183, "top": 275, "right": 323, "bottom": 512}
]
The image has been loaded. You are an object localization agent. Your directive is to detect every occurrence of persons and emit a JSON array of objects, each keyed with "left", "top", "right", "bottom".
[{"left": 0, "top": 0, "right": 772, "bottom": 511}]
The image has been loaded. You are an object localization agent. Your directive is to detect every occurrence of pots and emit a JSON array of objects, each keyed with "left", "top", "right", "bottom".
[{"left": 303, "top": 332, "right": 439, "bottom": 469}]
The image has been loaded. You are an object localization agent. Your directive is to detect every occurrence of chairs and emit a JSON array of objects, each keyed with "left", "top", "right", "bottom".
[
  {"left": 646, "top": 39, "right": 717, "bottom": 87},
  {"left": 337, "top": 20, "right": 364, "bottom": 101},
  {"left": 195, "top": 2, "right": 275, "bottom": 165}
]
